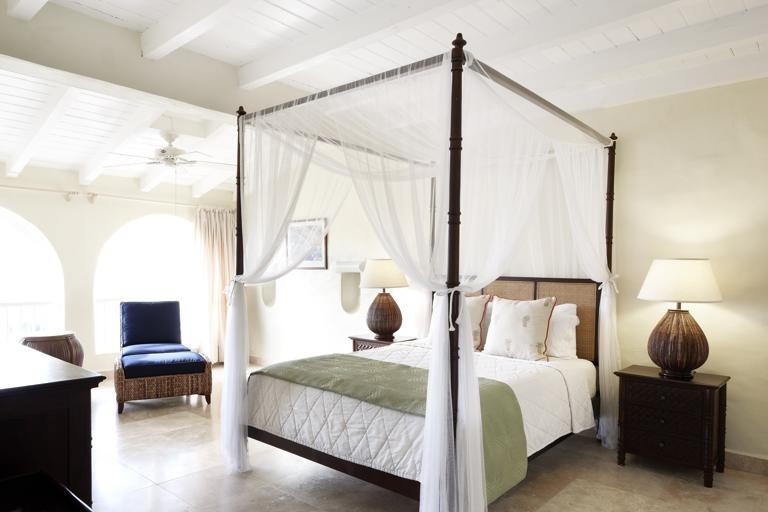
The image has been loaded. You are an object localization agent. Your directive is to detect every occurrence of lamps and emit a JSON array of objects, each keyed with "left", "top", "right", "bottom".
[
  {"left": 357, "top": 259, "right": 410, "bottom": 338},
  {"left": 636, "top": 258, "right": 722, "bottom": 381}
]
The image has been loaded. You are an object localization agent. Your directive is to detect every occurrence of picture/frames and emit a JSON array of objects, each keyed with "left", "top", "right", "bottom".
[{"left": 285, "top": 217, "right": 328, "bottom": 270}]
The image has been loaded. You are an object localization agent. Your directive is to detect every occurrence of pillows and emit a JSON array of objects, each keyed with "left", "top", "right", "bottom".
[
  {"left": 423, "top": 292, "right": 491, "bottom": 350},
  {"left": 483, "top": 295, "right": 556, "bottom": 361},
  {"left": 479, "top": 303, "right": 579, "bottom": 360}
]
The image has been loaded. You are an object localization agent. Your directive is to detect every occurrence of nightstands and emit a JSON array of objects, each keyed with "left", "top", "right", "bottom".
[
  {"left": 348, "top": 336, "right": 417, "bottom": 352},
  {"left": 614, "top": 363, "right": 731, "bottom": 490}
]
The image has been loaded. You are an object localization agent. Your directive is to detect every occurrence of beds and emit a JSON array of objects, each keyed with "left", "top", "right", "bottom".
[{"left": 236, "top": 271, "right": 620, "bottom": 512}]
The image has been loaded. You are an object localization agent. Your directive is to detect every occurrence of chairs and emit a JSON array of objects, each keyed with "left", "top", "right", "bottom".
[{"left": 113, "top": 301, "right": 212, "bottom": 415}]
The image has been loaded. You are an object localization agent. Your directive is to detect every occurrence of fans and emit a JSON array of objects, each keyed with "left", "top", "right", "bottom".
[{"left": 106, "top": 131, "right": 238, "bottom": 168}]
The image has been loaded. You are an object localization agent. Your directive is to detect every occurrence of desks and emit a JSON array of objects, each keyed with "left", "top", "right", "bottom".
[{"left": 0, "top": 341, "right": 107, "bottom": 512}]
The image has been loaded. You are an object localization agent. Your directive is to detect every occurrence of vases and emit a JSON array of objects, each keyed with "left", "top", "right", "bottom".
[{"left": 20, "top": 333, "right": 83, "bottom": 366}]
